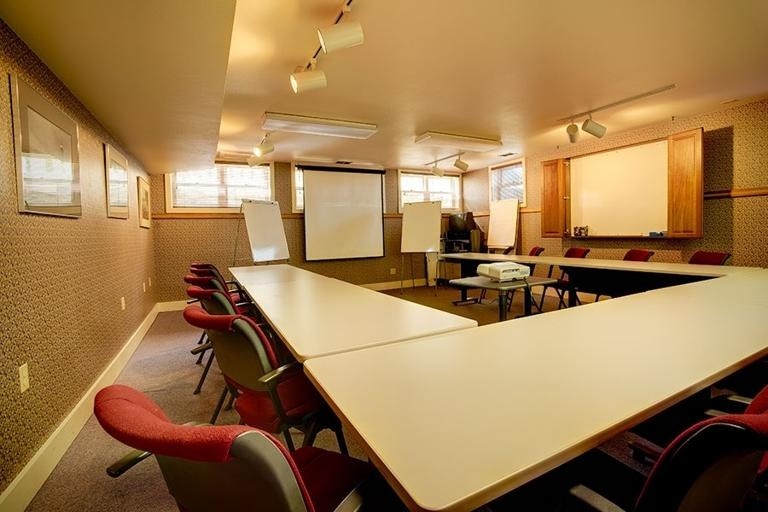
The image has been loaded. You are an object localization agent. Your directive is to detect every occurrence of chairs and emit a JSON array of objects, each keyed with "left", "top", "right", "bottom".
[
  {"left": 477, "top": 244, "right": 547, "bottom": 302},
  {"left": 686, "top": 249, "right": 732, "bottom": 266},
  {"left": 182, "top": 304, "right": 351, "bottom": 456},
  {"left": 537, "top": 246, "right": 591, "bottom": 311},
  {"left": 93, "top": 383, "right": 375, "bottom": 512},
  {"left": 181, "top": 260, "right": 259, "bottom": 365},
  {"left": 568, "top": 385, "right": 767, "bottom": 512},
  {"left": 594, "top": 247, "right": 655, "bottom": 305},
  {"left": 185, "top": 285, "right": 280, "bottom": 427}
]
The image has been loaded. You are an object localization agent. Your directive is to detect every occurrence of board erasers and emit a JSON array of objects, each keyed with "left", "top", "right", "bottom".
[{"left": 649, "top": 232, "right": 660, "bottom": 236}]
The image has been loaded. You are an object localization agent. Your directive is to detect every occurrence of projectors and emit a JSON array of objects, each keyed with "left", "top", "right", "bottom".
[{"left": 476, "top": 262, "right": 530, "bottom": 284}]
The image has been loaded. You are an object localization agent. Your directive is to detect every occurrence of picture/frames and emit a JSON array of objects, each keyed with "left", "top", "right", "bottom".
[
  {"left": 137, "top": 174, "right": 151, "bottom": 229},
  {"left": 10, "top": 69, "right": 82, "bottom": 222},
  {"left": 104, "top": 139, "right": 132, "bottom": 219}
]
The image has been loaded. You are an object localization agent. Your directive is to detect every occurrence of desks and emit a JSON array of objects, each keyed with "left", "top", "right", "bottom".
[
  {"left": 225, "top": 263, "right": 335, "bottom": 291},
  {"left": 438, "top": 250, "right": 763, "bottom": 306},
  {"left": 242, "top": 278, "right": 480, "bottom": 364},
  {"left": 303, "top": 266, "right": 768, "bottom": 511},
  {"left": 448, "top": 273, "right": 561, "bottom": 321}
]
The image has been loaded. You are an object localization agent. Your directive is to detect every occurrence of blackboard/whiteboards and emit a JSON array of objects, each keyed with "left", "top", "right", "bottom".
[
  {"left": 400, "top": 201, "right": 441, "bottom": 253},
  {"left": 243, "top": 199, "right": 290, "bottom": 262},
  {"left": 570, "top": 140, "right": 668, "bottom": 238},
  {"left": 487, "top": 199, "right": 518, "bottom": 247}
]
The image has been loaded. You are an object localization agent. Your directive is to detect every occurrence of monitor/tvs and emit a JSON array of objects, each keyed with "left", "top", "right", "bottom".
[{"left": 448, "top": 212, "right": 475, "bottom": 240}]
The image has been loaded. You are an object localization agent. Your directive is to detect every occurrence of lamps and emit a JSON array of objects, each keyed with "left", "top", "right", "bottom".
[
  {"left": 421, "top": 149, "right": 469, "bottom": 177},
  {"left": 557, "top": 81, "right": 680, "bottom": 146},
  {"left": 278, "top": 1, "right": 367, "bottom": 96},
  {"left": 258, "top": 110, "right": 378, "bottom": 140},
  {"left": 415, "top": 130, "right": 502, "bottom": 153},
  {"left": 247, "top": 128, "right": 276, "bottom": 168}
]
